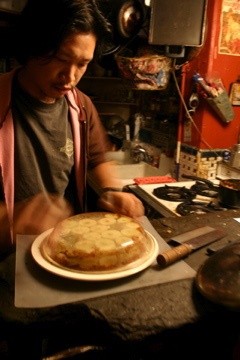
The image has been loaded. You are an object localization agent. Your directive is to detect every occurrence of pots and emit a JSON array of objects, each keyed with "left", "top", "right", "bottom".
[{"left": 181, "top": 173, "right": 240, "bottom": 210}]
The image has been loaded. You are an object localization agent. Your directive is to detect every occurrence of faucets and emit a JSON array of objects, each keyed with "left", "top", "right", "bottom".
[{"left": 134, "top": 149, "right": 148, "bottom": 163}]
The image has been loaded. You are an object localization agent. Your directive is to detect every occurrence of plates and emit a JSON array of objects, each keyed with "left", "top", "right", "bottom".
[{"left": 31, "top": 223, "right": 159, "bottom": 281}]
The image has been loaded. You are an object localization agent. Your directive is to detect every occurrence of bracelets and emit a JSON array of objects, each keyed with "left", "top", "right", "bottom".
[{"left": 99, "top": 187, "right": 119, "bottom": 194}]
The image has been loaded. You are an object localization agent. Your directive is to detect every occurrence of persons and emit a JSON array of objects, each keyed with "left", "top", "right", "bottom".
[{"left": 0, "top": 1, "right": 144, "bottom": 232}]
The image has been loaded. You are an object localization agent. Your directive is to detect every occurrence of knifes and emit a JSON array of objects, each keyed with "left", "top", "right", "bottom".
[
  {"left": 193, "top": 72, "right": 225, "bottom": 100},
  {"left": 157, "top": 225, "right": 228, "bottom": 267}
]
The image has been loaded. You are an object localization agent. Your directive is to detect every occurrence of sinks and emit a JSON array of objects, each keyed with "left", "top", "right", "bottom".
[{"left": 87, "top": 151, "right": 172, "bottom": 197}]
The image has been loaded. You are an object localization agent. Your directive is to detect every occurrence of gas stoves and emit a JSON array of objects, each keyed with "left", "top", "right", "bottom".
[{"left": 136, "top": 176, "right": 240, "bottom": 217}]
